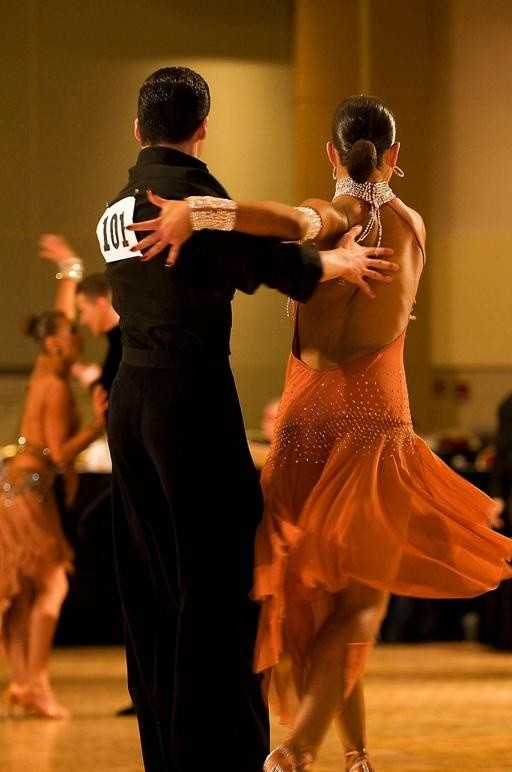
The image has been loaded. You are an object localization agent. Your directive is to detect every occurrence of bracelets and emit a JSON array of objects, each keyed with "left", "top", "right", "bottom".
[
  {"left": 59, "top": 257, "right": 85, "bottom": 285},
  {"left": 186, "top": 192, "right": 237, "bottom": 232}
]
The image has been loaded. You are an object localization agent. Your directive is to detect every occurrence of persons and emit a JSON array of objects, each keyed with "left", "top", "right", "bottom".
[
  {"left": 93, "top": 66, "right": 399, "bottom": 772},
  {"left": 58, "top": 272, "right": 141, "bottom": 719},
  {"left": 1, "top": 234, "right": 111, "bottom": 721},
  {"left": 126, "top": 91, "right": 512, "bottom": 772},
  {"left": 479, "top": 381, "right": 511, "bottom": 651}
]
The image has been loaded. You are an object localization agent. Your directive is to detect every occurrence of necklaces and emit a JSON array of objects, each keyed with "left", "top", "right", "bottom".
[{"left": 335, "top": 174, "right": 397, "bottom": 206}]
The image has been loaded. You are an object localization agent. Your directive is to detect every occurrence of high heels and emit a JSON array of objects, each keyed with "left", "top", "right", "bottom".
[{"left": 3, "top": 676, "right": 70, "bottom": 720}]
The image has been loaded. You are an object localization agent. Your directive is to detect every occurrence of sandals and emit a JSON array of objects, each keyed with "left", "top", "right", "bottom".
[{"left": 262, "top": 740, "right": 374, "bottom": 772}]
